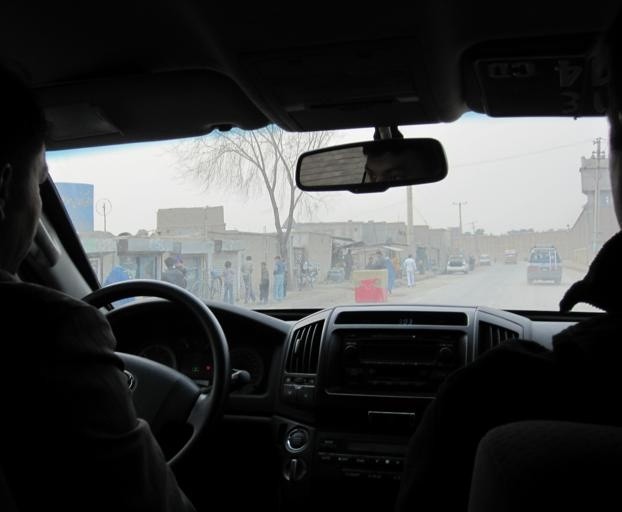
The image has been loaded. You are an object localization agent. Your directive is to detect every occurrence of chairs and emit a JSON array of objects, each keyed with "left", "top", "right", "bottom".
[{"left": 461, "top": 418, "right": 621, "bottom": 511}]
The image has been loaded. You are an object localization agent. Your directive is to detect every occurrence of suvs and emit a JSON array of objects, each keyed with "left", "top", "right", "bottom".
[{"left": 446, "top": 254, "right": 468, "bottom": 274}]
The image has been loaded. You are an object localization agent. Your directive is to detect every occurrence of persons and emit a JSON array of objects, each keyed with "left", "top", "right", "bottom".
[
  {"left": 102, "top": 253, "right": 190, "bottom": 294},
  {"left": 271, "top": 256, "right": 284, "bottom": 300},
  {"left": 332, "top": 248, "right": 401, "bottom": 294},
  {"left": 240, "top": 256, "right": 256, "bottom": 303},
  {"left": 363, "top": 138, "right": 443, "bottom": 184},
  {"left": 403, "top": 253, "right": 417, "bottom": 288},
  {"left": 221, "top": 260, "right": 236, "bottom": 305},
  {"left": 1, "top": 110, "right": 211, "bottom": 511},
  {"left": 394, "top": 68, "right": 621, "bottom": 511},
  {"left": 258, "top": 262, "right": 270, "bottom": 304}
]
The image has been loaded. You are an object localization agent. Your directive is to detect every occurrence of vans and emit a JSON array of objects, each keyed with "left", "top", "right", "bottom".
[
  {"left": 480, "top": 254, "right": 491, "bottom": 266},
  {"left": 526, "top": 246, "right": 563, "bottom": 286},
  {"left": 504, "top": 248, "right": 518, "bottom": 264}
]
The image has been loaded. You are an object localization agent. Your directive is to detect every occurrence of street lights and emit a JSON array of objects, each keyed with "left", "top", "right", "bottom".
[{"left": 454, "top": 200, "right": 468, "bottom": 235}]
